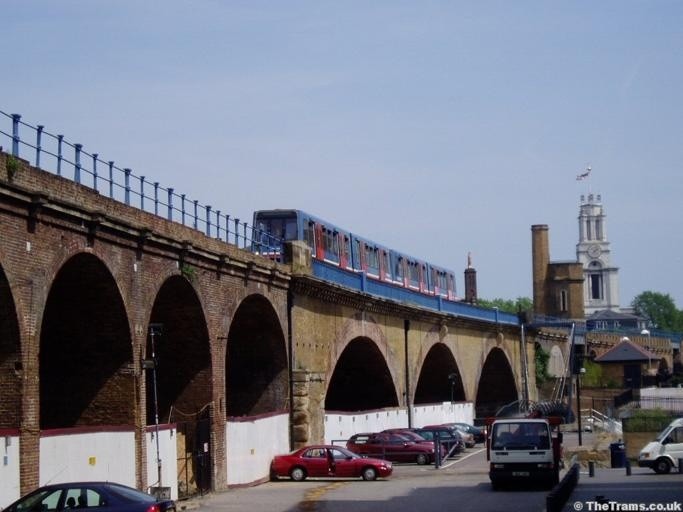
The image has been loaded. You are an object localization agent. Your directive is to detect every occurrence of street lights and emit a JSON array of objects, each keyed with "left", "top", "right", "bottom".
[
  {"left": 576, "top": 367, "right": 587, "bottom": 445},
  {"left": 641, "top": 329, "right": 652, "bottom": 368}
]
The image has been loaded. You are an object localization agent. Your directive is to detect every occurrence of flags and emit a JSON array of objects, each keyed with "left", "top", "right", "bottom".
[{"left": 575, "top": 173, "right": 588, "bottom": 181}]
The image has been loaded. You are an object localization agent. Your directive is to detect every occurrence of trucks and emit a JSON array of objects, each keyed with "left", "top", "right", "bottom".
[{"left": 487, "top": 417, "right": 562, "bottom": 490}]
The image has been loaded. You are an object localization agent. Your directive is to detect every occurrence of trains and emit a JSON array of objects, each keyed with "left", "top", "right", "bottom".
[{"left": 251, "top": 209, "right": 458, "bottom": 303}]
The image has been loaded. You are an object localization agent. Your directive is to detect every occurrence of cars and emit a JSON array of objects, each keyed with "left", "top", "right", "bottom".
[
  {"left": 0, "top": 481, "right": 176, "bottom": 512},
  {"left": 271, "top": 422, "right": 486, "bottom": 481}
]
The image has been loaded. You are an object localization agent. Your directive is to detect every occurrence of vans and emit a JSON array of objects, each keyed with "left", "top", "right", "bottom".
[{"left": 638, "top": 418, "right": 683, "bottom": 474}]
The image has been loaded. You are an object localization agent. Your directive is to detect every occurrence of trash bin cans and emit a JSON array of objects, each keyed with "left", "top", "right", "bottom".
[{"left": 608, "top": 442, "right": 627, "bottom": 467}]
